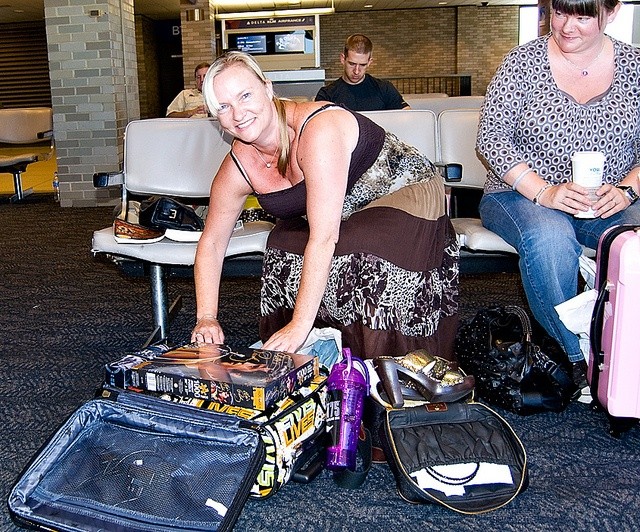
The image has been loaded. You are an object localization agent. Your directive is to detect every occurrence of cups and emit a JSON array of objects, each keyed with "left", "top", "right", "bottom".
[
  {"left": 570, "top": 150, "right": 605, "bottom": 220},
  {"left": 327, "top": 363, "right": 368, "bottom": 471}
]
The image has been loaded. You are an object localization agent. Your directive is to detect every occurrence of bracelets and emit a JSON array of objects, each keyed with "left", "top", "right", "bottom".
[
  {"left": 511, "top": 169, "right": 534, "bottom": 192},
  {"left": 532, "top": 184, "right": 553, "bottom": 205},
  {"left": 195, "top": 313, "right": 218, "bottom": 324}
]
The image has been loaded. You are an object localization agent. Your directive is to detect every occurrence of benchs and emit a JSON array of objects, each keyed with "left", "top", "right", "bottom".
[
  {"left": 60, "top": 111, "right": 516, "bottom": 347},
  {"left": 405, "top": 96, "right": 486, "bottom": 110},
  {"left": 0, "top": 106, "right": 52, "bottom": 201},
  {"left": 288, "top": 93, "right": 449, "bottom": 104}
]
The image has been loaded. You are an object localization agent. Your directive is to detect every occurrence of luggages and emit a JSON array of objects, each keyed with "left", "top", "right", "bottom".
[
  {"left": 6, "top": 342, "right": 331, "bottom": 532},
  {"left": 589, "top": 222, "right": 640, "bottom": 439}
]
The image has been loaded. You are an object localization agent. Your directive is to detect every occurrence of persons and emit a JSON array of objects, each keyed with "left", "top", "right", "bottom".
[
  {"left": 165, "top": 62, "right": 211, "bottom": 118},
  {"left": 189, "top": 51, "right": 458, "bottom": 367},
  {"left": 477, "top": 0, "right": 640, "bottom": 403},
  {"left": 315, "top": 34, "right": 413, "bottom": 111}
]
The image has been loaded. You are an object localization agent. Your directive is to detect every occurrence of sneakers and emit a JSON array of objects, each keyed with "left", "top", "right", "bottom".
[{"left": 566, "top": 361, "right": 594, "bottom": 404}]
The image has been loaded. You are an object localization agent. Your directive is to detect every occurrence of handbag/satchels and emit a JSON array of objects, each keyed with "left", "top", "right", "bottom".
[
  {"left": 452, "top": 305, "right": 582, "bottom": 414},
  {"left": 139, "top": 196, "right": 276, "bottom": 233},
  {"left": 379, "top": 401, "right": 530, "bottom": 514}
]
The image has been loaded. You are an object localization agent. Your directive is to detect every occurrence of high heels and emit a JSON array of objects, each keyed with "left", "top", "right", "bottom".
[{"left": 373, "top": 348, "right": 477, "bottom": 408}]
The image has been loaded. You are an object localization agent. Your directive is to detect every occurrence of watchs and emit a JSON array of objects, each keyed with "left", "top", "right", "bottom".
[{"left": 615, "top": 184, "right": 639, "bottom": 204}]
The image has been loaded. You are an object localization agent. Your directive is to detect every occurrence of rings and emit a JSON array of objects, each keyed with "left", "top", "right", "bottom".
[{"left": 197, "top": 333, "right": 204, "bottom": 338}]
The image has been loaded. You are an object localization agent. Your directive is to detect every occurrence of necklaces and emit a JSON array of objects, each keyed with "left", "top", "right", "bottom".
[{"left": 252, "top": 141, "right": 280, "bottom": 168}]
